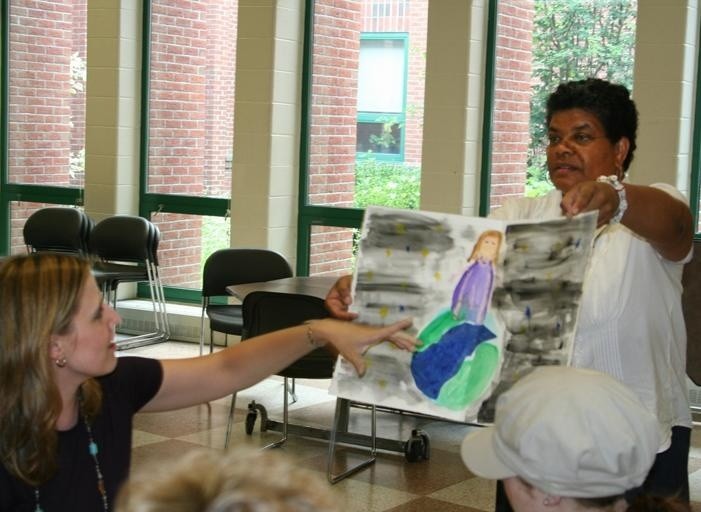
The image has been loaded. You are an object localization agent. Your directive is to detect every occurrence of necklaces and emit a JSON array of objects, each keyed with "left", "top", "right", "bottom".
[{"left": 22, "top": 390, "right": 111, "bottom": 512}]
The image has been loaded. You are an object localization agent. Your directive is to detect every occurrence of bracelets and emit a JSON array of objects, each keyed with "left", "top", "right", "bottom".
[
  {"left": 307, "top": 318, "right": 327, "bottom": 352},
  {"left": 599, "top": 175, "right": 629, "bottom": 228}
]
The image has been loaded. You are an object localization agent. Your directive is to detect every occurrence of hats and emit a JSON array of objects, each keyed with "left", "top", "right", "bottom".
[{"left": 460, "top": 365, "right": 662, "bottom": 500}]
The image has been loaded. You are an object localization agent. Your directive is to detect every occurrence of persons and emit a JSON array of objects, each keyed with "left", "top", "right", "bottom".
[
  {"left": 457, "top": 359, "right": 695, "bottom": 511},
  {"left": 0, "top": 251, "right": 425, "bottom": 512},
  {"left": 321, "top": 74, "right": 701, "bottom": 512},
  {"left": 112, "top": 442, "right": 341, "bottom": 511}
]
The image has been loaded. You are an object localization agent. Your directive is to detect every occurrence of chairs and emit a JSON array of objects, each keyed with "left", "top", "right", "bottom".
[
  {"left": 195, "top": 246, "right": 300, "bottom": 406},
  {"left": 219, "top": 287, "right": 379, "bottom": 485},
  {"left": 21, "top": 208, "right": 172, "bottom": 351}
]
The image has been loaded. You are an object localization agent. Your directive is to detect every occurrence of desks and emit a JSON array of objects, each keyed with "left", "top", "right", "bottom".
[{"left": 223, "top": 273, "right": 348, "bottom": 303}]
